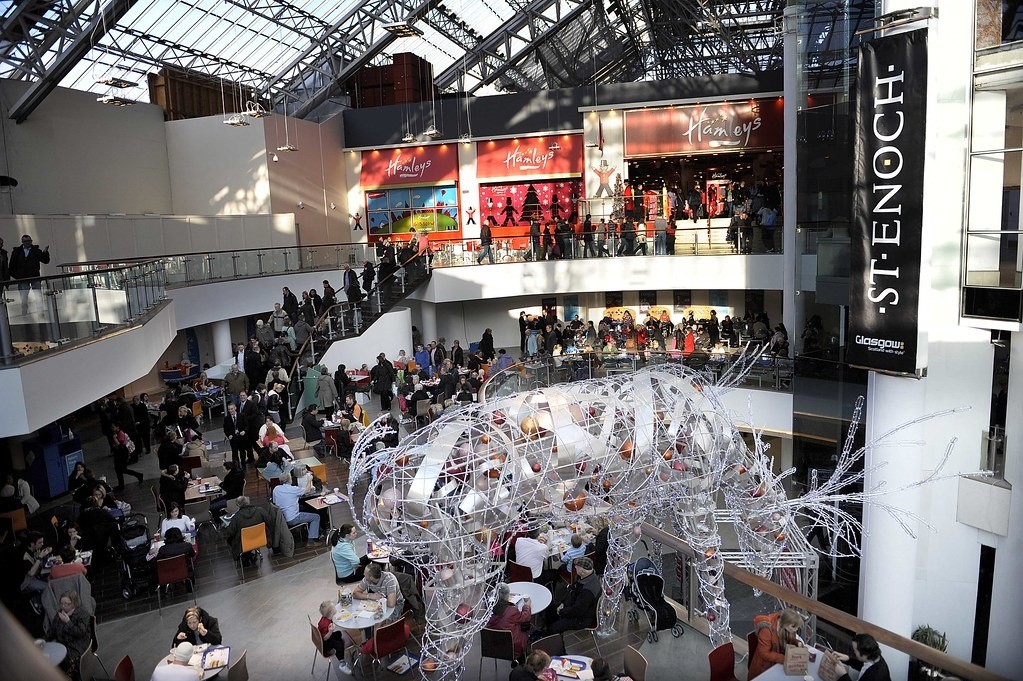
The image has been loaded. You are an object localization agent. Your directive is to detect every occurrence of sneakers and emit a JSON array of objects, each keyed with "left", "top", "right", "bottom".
[
  {"left": 29, "top": 598, "right": 43, "bottom": 615},
  {"left": 339, "top": 663, "right": 352, "bottom": 675}
]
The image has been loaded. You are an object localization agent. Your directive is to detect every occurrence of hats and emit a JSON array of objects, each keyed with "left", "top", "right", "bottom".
[
  {"left": 574, "top": 557, "right": 592, "bottom": 571},
  {"left": 175, "top": 642, "right": 194, "bottom": 661}
]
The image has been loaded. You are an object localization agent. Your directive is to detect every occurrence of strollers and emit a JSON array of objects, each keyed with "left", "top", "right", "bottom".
[
  {"left": 567, "top": 360, "right": 592, "bottom": 382},
  {"left": 107, "top": 513, "right": 156, "bottom": 597},
  {"left": 622, "top": 540, "right": 684, "bottom": 643}
]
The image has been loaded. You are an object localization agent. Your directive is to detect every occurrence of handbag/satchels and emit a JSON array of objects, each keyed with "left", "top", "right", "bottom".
[
  {"left": 784, "top": 643, "right": 809, "bottom": 676},
  {"left": 819, "top": 649, "right": 849, "bottom": 681},
  {"left": 333, "top": 398, "right": 341, "bottom": 411},
  {"left": 122, "top": 433, "right": 136, "bottom": 453}
]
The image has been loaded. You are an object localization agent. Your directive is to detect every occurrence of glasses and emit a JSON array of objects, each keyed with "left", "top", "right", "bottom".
[{"left": 60, "top": 602, "right": 72, "bottom": 607}]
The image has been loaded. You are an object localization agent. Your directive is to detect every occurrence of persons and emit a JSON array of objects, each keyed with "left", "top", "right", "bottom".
[
  {"left": 519, "top": 307, "right": 825, "bottom": 388},
  {"left": 623, "top": 179, "right": 781, "bottom": 255},
  {"left": 0, "top": 363, "right": 323, "bottom": 681},
  {"left": 0, "top": 235, "right": 50, "bottom": 320},
  {"left": 832, "top": 633, "right": 891, "bottom": 681},
  {"left": 796, "top": 485, "right": 834, "bottom": 556},
  {"left": 523, "top": 214, "right": 677, "bottom": 261},
  {"left": 318, "top": 478, "right": 633, "bottom": 681},
  {"left": 301, "top": 325, "right": 515, "bottom": 506},
  {"left": 747, "top": 608, "right": 803, "bottom": 681},
  {"left": 477, "top": 220, "right": 495, "bottom": 265},
  {"left": 232, "top": 227, "right": 434, "bottom": 401}
]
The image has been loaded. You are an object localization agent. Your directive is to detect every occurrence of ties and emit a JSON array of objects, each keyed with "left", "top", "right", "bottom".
[{"left": 233, "top": 415, "right": 236, "bottom": 430}]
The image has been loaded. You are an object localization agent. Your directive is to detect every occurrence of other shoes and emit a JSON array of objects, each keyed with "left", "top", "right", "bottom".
[
  {"left": 113, "top": 484, "right": 125, "bottom": 490},
  {"left": 241, "top": 464, "right": 247, "bottom": 473},
  {"left": 306, "top": 540, "right": 323, "bottom": 549},
  {"left": 138, "top": 473, "right": 144, "bottom": 484},
  {"left": 314, "top": 446, "right": 322, "bottom": 456}
]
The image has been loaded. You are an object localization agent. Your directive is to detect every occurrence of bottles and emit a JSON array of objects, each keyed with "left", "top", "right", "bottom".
[{"left": 338, "top": 586, "right": 352, "bottom": 607}]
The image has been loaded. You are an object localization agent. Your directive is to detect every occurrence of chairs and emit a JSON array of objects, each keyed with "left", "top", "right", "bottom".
[{"left": 0, "top": 309, "right": 897, "bottom": 681}]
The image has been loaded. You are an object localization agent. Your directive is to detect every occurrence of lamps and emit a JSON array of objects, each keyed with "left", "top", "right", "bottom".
[
  {"left": 241, "top": 19, "right": 270, "bottom": 119},
  {"left": 223, "top": 52, "right": 251, "bottom": 127},
  {"left": 377, "top": 0, "right": 423, "bottom": 38},
  {"left": 584, "top": 2, "right": 598, "bottom": 149},
  {"left": 423, "top": 0, "right": 443, "bottom": 140},
  {"left": 401, "top": 36, "right": 417, "bottom": 144},
  {"left": 95, "top": 16, "right": 137, "bottom": 107},
  {"left": 547, "top": 3, "right": 561, "bottom": 150},
  {"left": 90, "top": 1, "right": 140, "bottom": 88},
  {"left": 456, "top": 24, "right": 472, "bottom": 143},
  {"left": 277, "top": 21, "right": 298, "bottom": 153}
]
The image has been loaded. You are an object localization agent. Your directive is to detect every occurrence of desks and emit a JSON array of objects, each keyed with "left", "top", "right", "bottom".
[
  {"left": 407, "top": 391, "right": 433, "bottom": 401},
  {"left": 757, "top": 646, "right": 847, "bottom": 680},
  {"left": 318, "top": 420, "right": 351, "bottom": 431},
  {"left": 256, "top": 457, "right": 321, "bottom": 499},
  {"left": 184, "top": 479, "right": 223, "bottom": 500},
  {"left": 41, "top": 549, "right": 91, "bottom": 575},
  {"left": 220, "top": 511, "right": 236, "bottom": 526},
  {"left": 543, "top": 654, "right": 599, "bottom": 680},
  {"left": 500, "top": 580, "right": 552, "bottom": 616},
  {"left": 366, "top": 544, "right": 404, "bottom": 570},
  {"left": 537, "top": 521, "right": 597, "bottom": 568},
  {"left": 150, "top": 530, "right": 196, "bottom": 557},
  {"left": 517, "top": 362, "right": 551, "bottom": 389},
  {"left": 306, "top": 487, "right": 350, "bottom": 546},
  {"left": 152, "top": 641, "right": 228, "bottom": 679},
  {"left": 346, "top": 376, "right": 369, "bottom": 390},
  {"left": 333, "top": 592, "right": 397, "bottom": 627}
]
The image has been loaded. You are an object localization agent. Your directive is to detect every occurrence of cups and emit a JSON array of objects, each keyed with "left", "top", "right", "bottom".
[
  {"left": 803, "top": 675, "right": 814, "bottom": 681},
  {"left": 196, "top": 477, "right": 201, "bottom": 484},
  {"left": 379, "top": 598, "right": 387, "bottom": 614},
  {"left": 170, "top": 647, "right": 177, "bottom": 659},
  {"left": 332, "top": 415, "right": 337, "bottom": 422},
  {"left": 523, "top": 594, "right": 530, "bottom": 602},
  {"left": 205, "top": 482, "right": 209, "bottom": 490},
  {"left": 333, "top": 488, "right": 339, "bottom": 494},
  {"left": 154, "top": 533, "right": 161, "bottom": 543},
  {"left": 367, "top": 540, "right": 373, "bottom": 549},
  {"left": 808, "top": 649, "right": 817, "bottom": 662}
]
